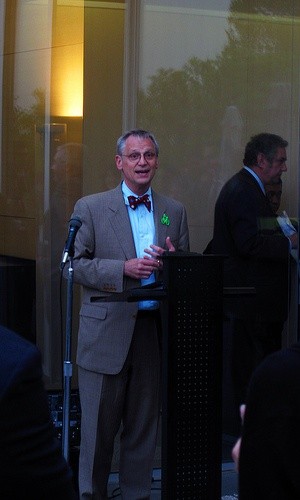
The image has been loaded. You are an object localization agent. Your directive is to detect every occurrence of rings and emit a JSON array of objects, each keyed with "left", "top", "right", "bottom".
[{"left": 157, "top": 260, "right": 160, "bottom": 264}]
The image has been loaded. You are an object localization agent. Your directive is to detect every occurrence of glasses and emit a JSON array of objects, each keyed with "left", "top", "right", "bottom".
[{"left": 120, "top": 152, "right": 156, "bottom": 160}]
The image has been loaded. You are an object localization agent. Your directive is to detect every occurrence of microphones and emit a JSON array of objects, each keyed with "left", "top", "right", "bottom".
[{"left": 61, "top": 216, "right": 82, "bottom": 268}]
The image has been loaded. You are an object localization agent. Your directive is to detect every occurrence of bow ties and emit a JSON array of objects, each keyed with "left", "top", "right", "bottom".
[{"left": 128, "top": 194, "right": 151, "bottom": 212}]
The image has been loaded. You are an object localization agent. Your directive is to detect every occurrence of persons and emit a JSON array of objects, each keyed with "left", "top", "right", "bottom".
[
  {"left": 60, "top": 129, "right": 190, "bottom": 500},
  {"left": 203, "top": 133, "right": 300, "bottom": 500},
  {"left": 0, "top": 323, "right": 75, "bottom": 500}
]
